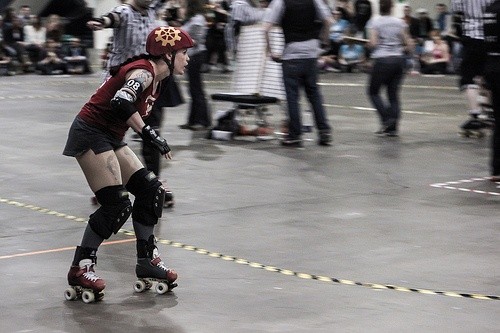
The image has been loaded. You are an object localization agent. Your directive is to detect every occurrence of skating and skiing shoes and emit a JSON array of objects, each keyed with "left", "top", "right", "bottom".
[
  {"left": 163, "top": 190, "right": 172, "bottom": 208},
  {"left": 134, "top": 236, "right": 178, "bottom": 295},
  {"left": 482, "top": 110, "right": 495, "bottom": 137},
  {"left": 64, "top": 247, "right": 105, "bottom": 304},
  {"left": 463, "top": 114, "right": 488, "bottom": 139}
]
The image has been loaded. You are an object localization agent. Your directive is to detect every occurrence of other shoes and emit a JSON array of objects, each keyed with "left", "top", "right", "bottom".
[
  {"left": 375, "top": 118, "right": 397, "bottom": 137},
  {"left": 180, "top": 123, "right": 191, "bottom": 130},
  {"left": 281, "top": 134, "right": 302, "bottom": 145}
]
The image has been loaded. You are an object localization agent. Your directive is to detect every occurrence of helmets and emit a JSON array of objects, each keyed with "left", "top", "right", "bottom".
[{"left": 145, "top": 25, "right": 193, "bottom": 56}]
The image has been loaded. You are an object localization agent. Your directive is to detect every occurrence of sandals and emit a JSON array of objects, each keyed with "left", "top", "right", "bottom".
[{"left": 319, "top": 130, "right": 330, "bottom": 145}]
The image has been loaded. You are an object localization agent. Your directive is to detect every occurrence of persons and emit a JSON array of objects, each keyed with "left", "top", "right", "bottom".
[
  {"left": 151, "top": 0, "right": 291, "bottom": 140},
  {"left": 402, "top": 3, "right": 464, "bottom": 75},
  {"left": 0, "top": 0, "right": 96, "bottom": 75},
  {"left": 319, "top": 0, "right": 373, "bottom": 74},
  {"left": 482, "top": 0, "right": 500, "bottom": 190},
  {"left": 453, "top": 0, "right": 493, "bottom": 89},
  {"left": 264, "top": 0, "right": 333, "bottom": 150},
  {"left": 61, "top": 26, "right": 193, "bottom": 302},
  {"left": 88, "top": 0, "right": 174, "bottom": 210},
  {"left": 366, "top": 0, "right": 415, "bottom": 137}
]
